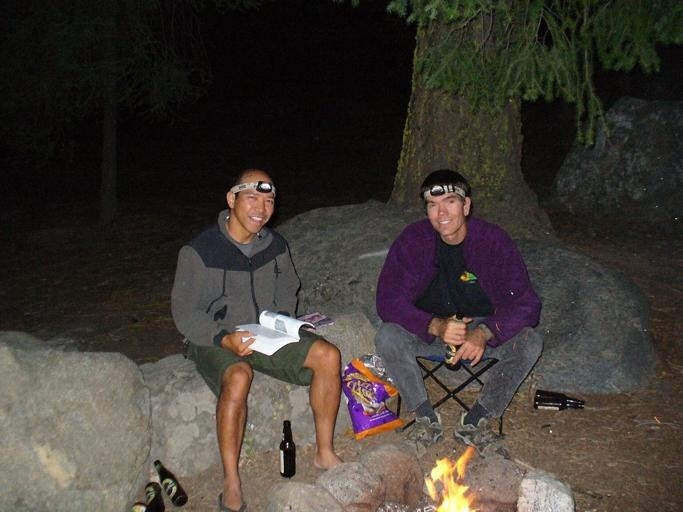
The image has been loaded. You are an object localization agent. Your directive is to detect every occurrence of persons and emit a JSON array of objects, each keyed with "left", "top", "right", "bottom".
[
  {"left": 373, "top": 169, "right": 544, "bottom": 459},
  {"left": 170, "top": 168, "right": 346, "bottom": 512}
]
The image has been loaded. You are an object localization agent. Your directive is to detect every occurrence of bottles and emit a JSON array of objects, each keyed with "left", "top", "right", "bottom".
[
  {"left": 533, "top": 389, "right": 584, "bottom": 404},
  {"left": 145, "top": 481, "right": 166, "bottom": 512},
  {"left": 131, "top": 502, "right": 147, "bottom": 512},
  {"left": 153, "top": 460, "right": 188, "bottom": 505},
  {"left": 533, "top": 396, "right": 585, "bottom": 412},
  {"left": 278, "top": 419, "right": 297, "bottom": 479},
  {"left": 445, "top": 313, "right": 465, "bottom": 371}
]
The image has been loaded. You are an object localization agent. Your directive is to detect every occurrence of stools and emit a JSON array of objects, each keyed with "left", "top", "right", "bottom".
[{"left": 393, "top": 354, "right": 503, "bottom": 438}]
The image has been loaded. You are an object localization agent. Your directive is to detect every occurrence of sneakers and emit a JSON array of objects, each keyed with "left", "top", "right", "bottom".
[
  {"left": 453, "top": 412, "right": 512, "bottom": 461},
  {"left": 405, "top": 410, "right": 445, "bottom": 458}
]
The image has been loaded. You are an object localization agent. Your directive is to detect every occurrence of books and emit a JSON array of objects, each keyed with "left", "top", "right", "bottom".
[
  {"left": 234, "top": 309, "right": 316, "bottom": 357},
  {"left": 297, "top": 311, "right": 335, "bottom": 332}
]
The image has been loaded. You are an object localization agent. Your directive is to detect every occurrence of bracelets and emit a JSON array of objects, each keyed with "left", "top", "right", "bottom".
[{"left": 478, "top": 323, "right": 492, "bottom": 340}]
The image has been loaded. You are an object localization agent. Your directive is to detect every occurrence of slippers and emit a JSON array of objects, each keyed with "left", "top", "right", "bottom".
[{"left": 216, "top": 490, "right": 248, "bottom": 511}]
